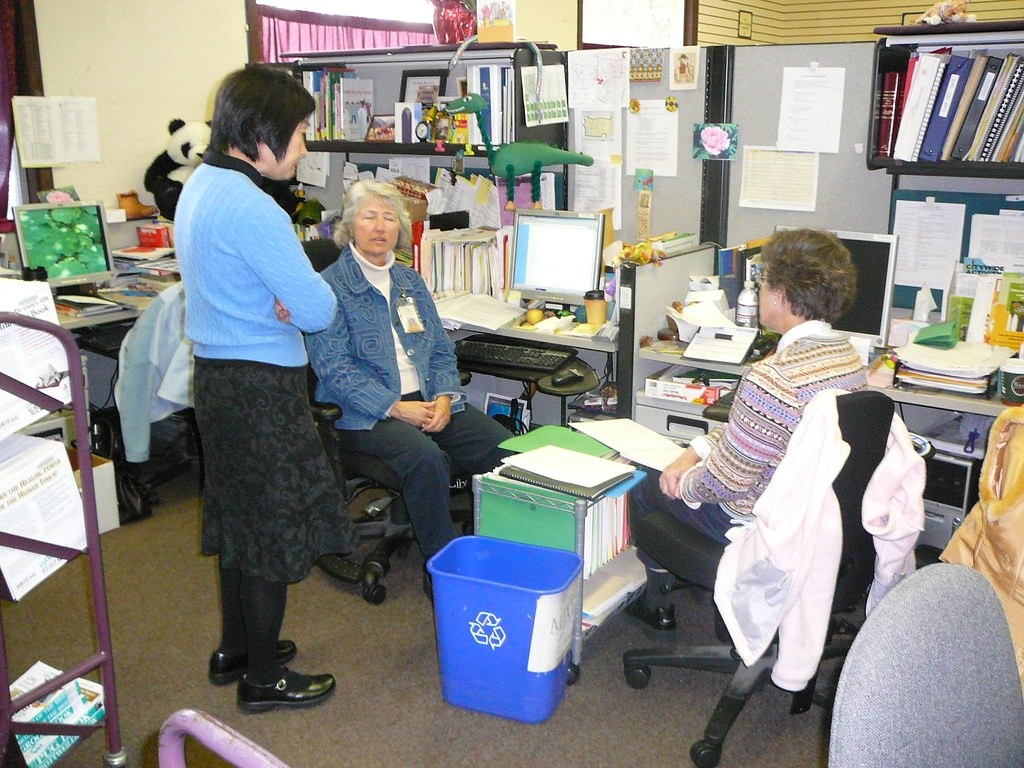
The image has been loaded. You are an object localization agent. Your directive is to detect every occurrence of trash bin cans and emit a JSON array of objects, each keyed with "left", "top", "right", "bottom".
[{"left": 427, "top": 534, "right": 583, "bottom": 727}]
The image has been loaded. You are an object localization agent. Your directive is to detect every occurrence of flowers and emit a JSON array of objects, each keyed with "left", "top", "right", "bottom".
[
  {"left": 37, "top": 185, "right": 80, "bottom": 203},
  {"left": 693, "top": 123, "right": 738, "bottom": 161}
]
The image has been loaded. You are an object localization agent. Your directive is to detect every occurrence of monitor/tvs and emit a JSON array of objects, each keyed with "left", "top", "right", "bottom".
[
  {"left": 12, "top": 200, "right": 116, "bottom": 289},
  {"left": 509, "top": 208, "right": 606, "bottom": 305},
  {"left": 774, "top": 226, "right": 899, "bottom": 348}
]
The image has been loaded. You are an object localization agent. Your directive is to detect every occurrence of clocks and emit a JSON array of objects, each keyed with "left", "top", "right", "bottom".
[{"left": 415, "top": 121, "right": 430, "bottom": 143}]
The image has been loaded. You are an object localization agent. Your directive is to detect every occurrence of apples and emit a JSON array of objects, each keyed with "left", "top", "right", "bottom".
[{"left": 527, "top": 309, "right": 543, "bottom": 324}]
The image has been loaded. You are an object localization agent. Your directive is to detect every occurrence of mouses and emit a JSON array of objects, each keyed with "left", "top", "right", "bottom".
[{"left": 550, "top": 368, "right": 584, "bottom": 386}]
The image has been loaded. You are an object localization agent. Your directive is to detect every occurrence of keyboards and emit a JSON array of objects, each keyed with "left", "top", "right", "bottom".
[
  {"left": 453, "top": 340, "right": 573, "bottom": 370},
  {"left": 76, "top": 324, "right": 133, "bottom": 353}
]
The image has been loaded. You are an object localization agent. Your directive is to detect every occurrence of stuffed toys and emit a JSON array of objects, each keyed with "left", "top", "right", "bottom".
[
  {"left": 914, "top": 0, "right": 976, "bottom": 25},
  {"left": 144, "top": 118, "right": 213, "bottom": 221}
]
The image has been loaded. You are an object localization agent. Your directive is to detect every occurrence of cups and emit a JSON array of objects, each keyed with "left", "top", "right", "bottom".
[
  {"left": 583, "top": 290, "right": 607, "bottom": 326},
  {"left": 999, "top": 358, "right": 1024, "bottom": 407}
]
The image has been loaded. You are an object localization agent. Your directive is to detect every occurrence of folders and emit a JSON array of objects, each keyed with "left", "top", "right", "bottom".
[
  {"left": 918, "top": 54, "right": 975, "bottom": 163},
  {"left": 951, "top": 56, "right": 1004, "bottom": 159}
]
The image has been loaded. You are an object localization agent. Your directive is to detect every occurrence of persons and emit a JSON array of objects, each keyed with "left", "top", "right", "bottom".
[
  {"left": 302, "top": 179, "right": 516, "bottom": 607},
  {"left": 619, "top": 228, "right": 867, "bottom": 631},
  {"left": 173, "top": 63, "right": 355, "bottom": 715}
]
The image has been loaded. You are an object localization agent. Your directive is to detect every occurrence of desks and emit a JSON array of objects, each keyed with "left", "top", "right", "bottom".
[
  {"left": 634, "top": 340, "right": 1015, "bottom": 462},
  {"left": 57, "top": 307, "right": 147, "bottom": 329},
  {"left": 440, "top": 321, "right": 620, "bottom": 353}
]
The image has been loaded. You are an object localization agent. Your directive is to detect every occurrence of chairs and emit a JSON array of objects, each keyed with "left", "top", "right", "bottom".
[
  {"left": 113, "top": 281, "right": 205, "bottom": 520},
  {"left": 827, "top": 562, "right": 1024, "bottom": 768},
  {"left": 623, "top": 388, "right": 895, "bottom": 768},
  {"left": 300, "top": 239, "right": 474, "bottom": 605}
]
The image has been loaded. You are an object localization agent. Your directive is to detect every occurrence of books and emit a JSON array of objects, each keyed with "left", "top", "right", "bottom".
[
  {"left": 9, "top": 660, "right": 105, "bottom": 768},
  {"left": 287, "top": 66, "right": 374, "bottom": 142},
  {"left": 53, "top": 222, "right": 181, "bottom": 317},
  {"left": 480, "top": 424, "right": 647, "bottom": 633},
  {"left": 892, "top": 257, "right": 1024, "bottom": 394},
  {"left": 673, "top": 368, "right": 740, "bottom": 391},
  {"left": 874, "top": 44, "right": 1024, "bottom": 163},
  {"left": 684, "top": 245, "right": 763, "bottom": 312},
  {"left": 420, "top": 226, "right": 512, "bottom": 297},
  {"left": 467, "top": 65, "right": 515, "bottom": 144},
  {"left": 294, "top": 223, "right": 320, "bottom": 242}
]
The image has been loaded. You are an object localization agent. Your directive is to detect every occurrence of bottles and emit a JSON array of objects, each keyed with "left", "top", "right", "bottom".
[{"left": 736, "top": 281, "right": 763, "bottom": 329}]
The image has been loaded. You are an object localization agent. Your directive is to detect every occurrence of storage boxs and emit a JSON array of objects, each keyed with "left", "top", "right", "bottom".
[
  {"left": 66, "top": 446, "right": 120, "bottom": 536},
  {"left": 395, "top": 102, "right": 422, "bottom": 143},
  {"left": 478, "top": 25, "right": 513, "bottom": 42},
  {"left": 393, "top": 176, "right": 438, "bottom": 223},
  {"left": 644, "top": 366, "right": 725, "bottom": 406}
]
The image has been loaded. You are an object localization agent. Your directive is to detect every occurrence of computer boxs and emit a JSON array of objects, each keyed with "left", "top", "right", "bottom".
[{"left": 916, "top": 450, "right": 982, "bottom": 551}]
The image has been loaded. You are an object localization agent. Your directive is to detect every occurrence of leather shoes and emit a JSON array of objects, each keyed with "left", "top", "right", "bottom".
[
  {"left": 235, "top": 664, "right": 337, "bottom": 714},
  {"left": 209, "top": 639, "right": 297, "bottom": 686}
]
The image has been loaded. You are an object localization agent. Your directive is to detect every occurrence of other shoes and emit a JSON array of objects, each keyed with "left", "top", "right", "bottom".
[
  {"left": 421, "top": 568, "right": 434, "bottom": 601},
  {"left": 626, "top": 600, "right": 675, "bottom": 631}
]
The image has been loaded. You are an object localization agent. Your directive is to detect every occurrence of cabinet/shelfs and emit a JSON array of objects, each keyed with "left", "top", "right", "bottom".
[
  {"left": 281, "top": 43, "right": 568, "bottom": 158},
  {"left": 0, "top": 311, "right": 126, "bottom": 768},
  {"left": 867, "top": 20, "right": 1024, "bottom": 180},
  {"left": 469, "top": 429, "right": 700, "bottom": 686}
]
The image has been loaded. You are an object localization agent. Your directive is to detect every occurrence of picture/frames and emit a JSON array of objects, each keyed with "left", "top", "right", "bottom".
[
  {"left": 431, "top": 116, "right": 452, "bottom": 143},
  {"left": 364, "top": 114, "right": 395, "bottom": 142}
]
